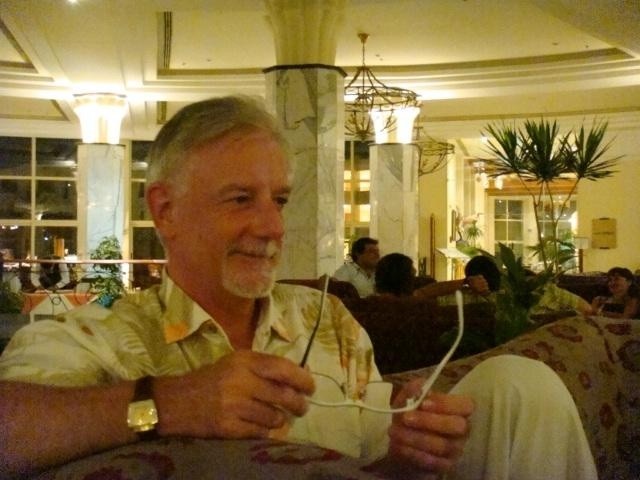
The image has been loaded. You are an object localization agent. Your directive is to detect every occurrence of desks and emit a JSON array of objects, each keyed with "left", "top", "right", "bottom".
[{"left": 20, "top": 291, "right": 98, "bottom": 323}]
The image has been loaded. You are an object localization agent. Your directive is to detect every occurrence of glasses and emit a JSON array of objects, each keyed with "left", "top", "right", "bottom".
[{"left": 279, "top": 273, "right": 468, "bottom": 415}]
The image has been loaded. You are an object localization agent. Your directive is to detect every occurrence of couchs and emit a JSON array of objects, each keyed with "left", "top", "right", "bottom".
[
  {"left": 24, "top": 315, "right": 640, "bottom": 479},
  {"left": 276, "top": 280, "right": 497, "bottom": 374},
  {"left": 544, "top": 273, "right": 640, "bottom": 321}
]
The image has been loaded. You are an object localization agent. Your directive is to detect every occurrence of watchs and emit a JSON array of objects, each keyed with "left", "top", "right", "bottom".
[
  {"left": 462, "top": 276, "right": 471, "bottom": 289},
  {"left": 124, "top": 374, "right": 160, "bottom": 443}
]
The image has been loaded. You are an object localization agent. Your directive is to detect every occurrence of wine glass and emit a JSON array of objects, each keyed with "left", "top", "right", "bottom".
[{"left": 596, "top": 296, "right": 606, "bottom": 315}]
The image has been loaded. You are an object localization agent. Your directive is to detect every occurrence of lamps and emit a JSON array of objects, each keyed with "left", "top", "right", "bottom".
[
  {"left": 368, "top": 100, "right": 423, "bottom": 145},
  {"left": 68, "top": 91, "right": 130, "bottom": 146},
  {"left": 345, "top": 34, "right": 417, "bottom": 143},
  {"left": 573, "top": 236, "right": 588, "bottom": 273},
  {"left": 414, "top": 102, "right": 454, "bottom": 176}
]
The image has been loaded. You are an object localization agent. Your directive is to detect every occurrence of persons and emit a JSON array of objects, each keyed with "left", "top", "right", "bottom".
[
  {"left": 0, "top": 93, "right": 599, "bottom": 479},
  {"left": 331, "top": 236, "right": 380, "bottom": 298},
  {"left": 413, "top": 255, "right": 591, "bottom": 315},
  {"left": 592, "top": 266, "right": 638, "bottom": 318},
  {"left": 29, "top": 254, "right": 70, "bottom": 290},
  {"left": 372, "top": 251, "right": 417, "bottom": 297}
]
